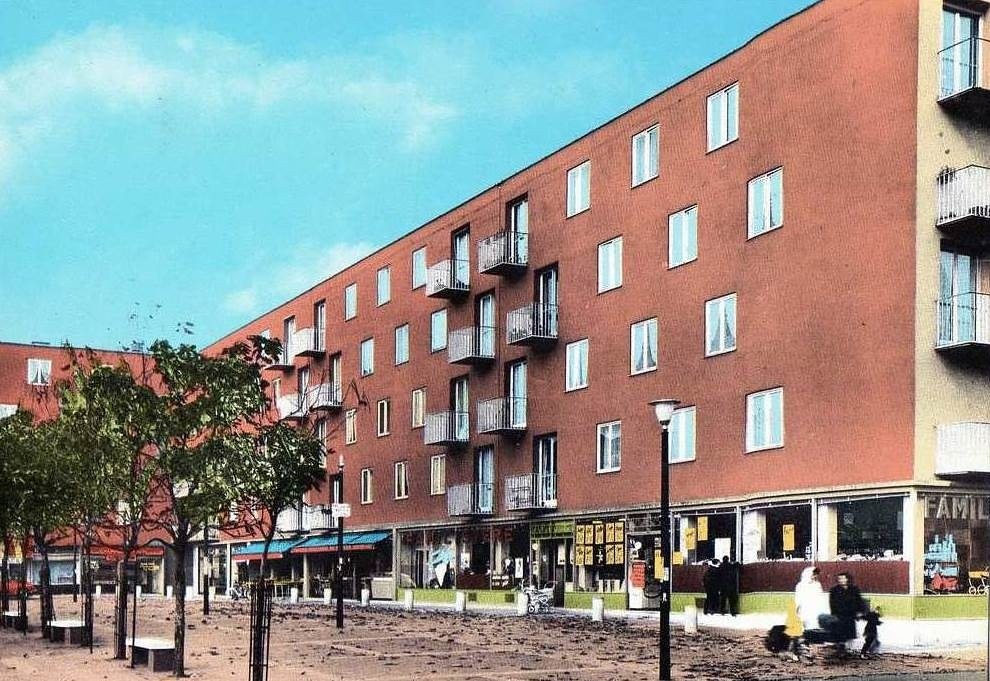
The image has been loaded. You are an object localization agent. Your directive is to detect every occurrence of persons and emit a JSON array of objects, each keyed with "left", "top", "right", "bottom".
[
  {"left": 829, "top": 572, "right": 880, "bottom": 661},
  {"left": 715, "top": 556, "right": 738, "bottom": 617},
  {"left": 729, "top": 556, "right": 741, "bottom": 575},
  {"left": 794, "top": 565, "right": 845, "bottom": 662},
  {"left": 704, "top": 558, "right": 721, "bottom": 615}
]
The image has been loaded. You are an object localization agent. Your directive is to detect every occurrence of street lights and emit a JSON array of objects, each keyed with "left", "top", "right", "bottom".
[{"left": 647, "top": 399, "right": 683, "bottom": 681}]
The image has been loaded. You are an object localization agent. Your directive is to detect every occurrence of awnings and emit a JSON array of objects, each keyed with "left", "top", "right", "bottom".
[
  {"left": 230, "top": 538, "right": 305, "bottom": 560},
  {"left": 289, "top": 530, "right": 389, "bottom": 554}
]
